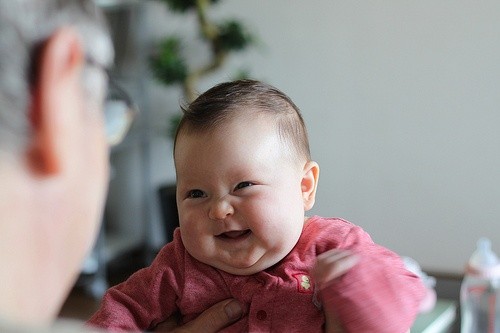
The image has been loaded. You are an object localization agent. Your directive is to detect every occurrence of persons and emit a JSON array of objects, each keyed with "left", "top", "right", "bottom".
[
  {"left": 90, "top": 82, "right": 443, "bottom": 333},
  {"left": 1, "top": 0, "right": 130, "bottom": 333}
]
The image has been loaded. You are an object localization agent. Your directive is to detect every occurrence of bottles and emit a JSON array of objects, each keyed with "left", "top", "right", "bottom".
[{"left": 459, "top": 238, "right": 499, "bottom": 333}]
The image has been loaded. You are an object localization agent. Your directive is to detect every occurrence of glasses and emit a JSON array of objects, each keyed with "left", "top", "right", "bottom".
[{"left": 83, "top": 56, "right": 138, "bottom": 149}]
